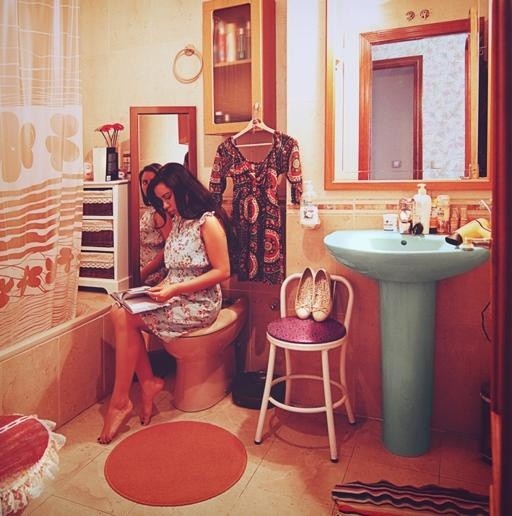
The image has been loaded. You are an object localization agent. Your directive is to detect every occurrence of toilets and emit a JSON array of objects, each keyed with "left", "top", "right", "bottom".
[{"left": 162, "top": 295, "right": 250, "bottom": 413}]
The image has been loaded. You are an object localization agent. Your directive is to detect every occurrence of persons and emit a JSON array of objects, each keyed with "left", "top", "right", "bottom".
[
  {"left": 138, "top": 163, "right": 172, "bottom": 286},
  {"left": 96, "top": 161, "right": 232, "bottom": 446}
]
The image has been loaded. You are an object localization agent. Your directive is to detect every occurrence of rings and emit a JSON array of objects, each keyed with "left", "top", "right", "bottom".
[{"left": 154, "top": 297, "right": 159, "bottom": 303}]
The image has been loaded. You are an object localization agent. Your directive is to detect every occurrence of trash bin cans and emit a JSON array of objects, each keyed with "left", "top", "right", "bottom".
[{"left": 479, "top": 391, "right": 491, "bottom": 464}]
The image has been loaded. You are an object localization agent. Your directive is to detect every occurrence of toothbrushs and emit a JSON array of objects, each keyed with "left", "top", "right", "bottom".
[{"left": 479, "top": 200, "right": 491, "bottom": 214}]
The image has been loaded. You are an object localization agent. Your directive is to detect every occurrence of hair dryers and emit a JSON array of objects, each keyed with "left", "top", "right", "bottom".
[{"left": 445, "top": 218, "right": 491, "bottom": 246}]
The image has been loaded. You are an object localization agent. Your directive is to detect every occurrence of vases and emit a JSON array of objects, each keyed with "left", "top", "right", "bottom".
[{"left": 106, "top": 148, "right": 118, "bottom": 181}]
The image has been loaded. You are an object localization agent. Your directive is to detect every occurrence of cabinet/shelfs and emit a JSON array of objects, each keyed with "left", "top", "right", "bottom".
[
  {"left": 202, "top": 0, "right": 275, "bottom": 135},
  {"left": 79, "top": 182, "right": 129, "bottom": 295}
]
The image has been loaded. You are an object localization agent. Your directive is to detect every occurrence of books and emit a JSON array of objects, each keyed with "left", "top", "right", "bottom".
[{"left": 109, "top": 285, "right": 181, "bottom": 315}]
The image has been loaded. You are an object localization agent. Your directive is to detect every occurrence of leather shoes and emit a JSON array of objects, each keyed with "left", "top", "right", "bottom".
[
  {"left": 311, "top": 267, "right": 334, "bottom": 324},
  {"left": 294, "top": 267, "right": 315, "bottom": 321}
]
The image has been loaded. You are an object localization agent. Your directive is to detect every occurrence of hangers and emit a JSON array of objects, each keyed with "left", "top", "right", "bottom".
[{"left": 224, "top": 103, "right": 278, "bottom": 148}]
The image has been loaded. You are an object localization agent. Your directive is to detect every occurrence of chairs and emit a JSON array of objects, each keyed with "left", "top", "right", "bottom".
[{"left": 255, "top": 272, "right": 357, "bottom": 464}]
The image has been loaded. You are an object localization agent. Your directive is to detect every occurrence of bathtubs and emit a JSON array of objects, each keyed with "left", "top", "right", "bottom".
[{"left": 0, "top": 291, "right": 117, "bottom": 432}]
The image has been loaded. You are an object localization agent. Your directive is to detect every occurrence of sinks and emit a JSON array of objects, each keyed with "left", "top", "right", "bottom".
[{"left": 323, "top": 228, "right": 491, "bottom": 284}]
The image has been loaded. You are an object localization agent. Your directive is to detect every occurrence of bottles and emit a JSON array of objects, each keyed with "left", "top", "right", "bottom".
[
  {"left": 224, "top": 113, "right": 230, "bottom": 122},
  {"left": 430, "top": 209, "right": 439, "bottom": 232},
  {"left": 449, "top": 206, "right": 460, "bottom": 232},
  {"left": 122, "top": 150, "right": 131, "bottom": 174},
  {"left": 214, "top": 111, "right": 224, "bottom": 124},
  {"left": 460, "top": 206, "right": 469, "bottom": 227},
  {"left": 214, "top": 16, "right": 251, "bottom": 63},
  {"left": 84, "top": 149, "right": 94, "bottom": 182},
  {"left": 438, "top": 209, "right": 447, "bottom": 233}
]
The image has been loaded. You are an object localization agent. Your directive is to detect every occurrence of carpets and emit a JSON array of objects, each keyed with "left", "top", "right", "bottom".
[
  {"left": 103, "top": 421, "right": 246, "bottom": 507},
  {"left": 333, "top": 480, "right": 512, "bottom": 516}
]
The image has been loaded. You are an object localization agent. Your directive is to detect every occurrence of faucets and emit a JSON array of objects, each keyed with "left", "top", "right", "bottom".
[{"left": 397, "top": 207, "right": 413, "bottom": 234}]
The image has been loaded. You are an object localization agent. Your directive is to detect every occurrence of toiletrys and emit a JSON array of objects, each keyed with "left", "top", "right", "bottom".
[
  {"left": 211, "top": 15, "right": 251, "bottom": 65},
  {"left": 459, "top": 204, "right": 469, "bottom": 230},
  {"left": 382, "top": 203, "right": 400, "bottom": 231},
  {"left": 449, "top": 204, "right": 459, "bottom": 234},
  {"left": 429, "top": 197, "right": 438, "bottom": 234},
  {"left": 412, "top": 183, "right": 432, "bottom": 235},
  {"left": 437, "top": 195, "right": 450, "bottom": 233}
]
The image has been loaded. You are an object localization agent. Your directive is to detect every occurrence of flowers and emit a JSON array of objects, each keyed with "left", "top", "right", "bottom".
[{"left": 95, "top": 123, "right": 123, "bottom": 147}]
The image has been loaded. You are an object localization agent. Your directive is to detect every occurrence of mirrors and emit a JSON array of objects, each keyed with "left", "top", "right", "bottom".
[
  {"left": 325, "top": 0, "right": 493, "bottom": 192},
  {"left": 131, "top": 105, "right": 197, "bottom": 296}
]
